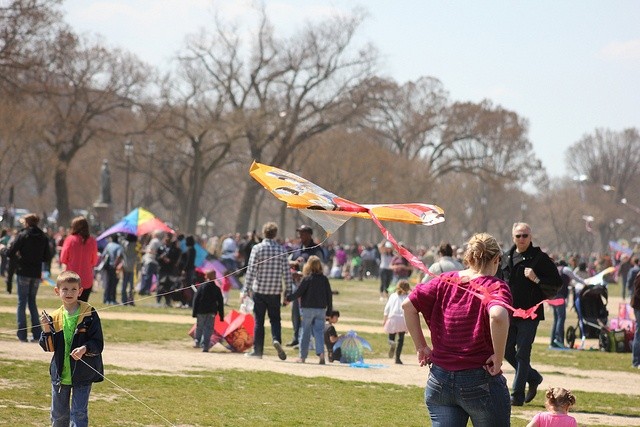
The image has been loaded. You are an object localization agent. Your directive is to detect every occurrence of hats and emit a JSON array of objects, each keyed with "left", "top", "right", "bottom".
[{"left": 296, "top": 224, "right": 312, "bottom": 234}]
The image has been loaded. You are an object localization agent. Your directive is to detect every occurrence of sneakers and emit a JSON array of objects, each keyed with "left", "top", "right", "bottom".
[
  {"left": 272, "top": 339, "right": 286, "bottom": 360},
  {"left": 320, "top": 355, "right": 326, "bottom": 364},
  {"left": 244, "top": 351, "right": 263, "bottom": 357}
]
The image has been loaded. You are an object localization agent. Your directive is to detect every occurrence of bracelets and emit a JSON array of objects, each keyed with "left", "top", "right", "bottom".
[{"left": 534, "top": 276, "right": 537, "bottom": 283}]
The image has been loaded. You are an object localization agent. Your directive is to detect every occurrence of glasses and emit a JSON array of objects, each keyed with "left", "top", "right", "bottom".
[{"left": 514, "top": 234, "right": 529, "bottom": 238}]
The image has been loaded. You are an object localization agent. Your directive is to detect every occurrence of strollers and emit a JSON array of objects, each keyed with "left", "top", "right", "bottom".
[{"left": 565, "top": 282, "right": 612, "bottom": 351}]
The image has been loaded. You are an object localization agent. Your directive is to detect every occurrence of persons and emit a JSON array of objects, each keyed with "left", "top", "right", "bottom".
[
  {"left": 572, "top": 263, "right": 589, "bottom": 311},
  {"left": 402, "top": 233, "right": 511, "bottom": 427},
  {"left": 390, "top": 241, "right": 413, "bottom": 287},
  {"left": 321, "top": 240, "right": 381, "bottom": 281},
  {"left": 284, "top": 255, "right": 332, "bottom": 365},
  {"left": 0, "top": 213, "right": 50, "bottom": 343},
  {"left": 421, "top": 242, "right": 465, "bottom": 284},
  {"left": 617, "top": 253, "right": 635, "bottom": 300},
  {"left": 100, "top": 234, "right": 123, "bottom": 305},
  {"left": 239, "top": 222, "right": 292, "bottom": 361},
  {"left": 378, "top": 238, "right": 395, "bottom": 301},
  {"left": 549, "top": 252, "right": 590, "bottom": 267},
  {"left": 120, "top": 233, "right": 137, "bottom": 306},
  {"left": 590, "top": 252, "right": 612, "bottom": 281},
  {"left": 136, "top": 229, "right": 196, "bottom": 308},
  {"left": 630, "top": 271, "right": 640, "bottom": 369},
  {"left": 286, "top": 225, "right": 325, "bottom": 351},
  {"left": 382, "top": 280, "right": 412, "bottom": 364},
  {"left": 0, "top": 229, "right": 20, "bottom": 295},
  {"left": 42, "top": 223, "right": 66, "bottom": 271},
  {"left": 548, "top": 260, "right": 587, "bottom": 348},
  {"left": 627, "top": 258, "right": 640, "bottom": 302},
  {"left": 323, "top": 311, "right": 345, "bottom": 363},
  {"left": 191, "top": 269, "right": 223, "bottom": 352},
  {"left": 195, "top": 230, "right": 262, "bottom": 277},
  {"left": 495, "top": 222, "right": 563, "bottom": 406},
  {"left": 39, "top": 270, "right": 103, "bottom": 427},
  {"left": 61, "top": 216, "right": 100, "bottom": 303},
  {"left": 526, "top": 387, "right": 578, "bottom": 427}
]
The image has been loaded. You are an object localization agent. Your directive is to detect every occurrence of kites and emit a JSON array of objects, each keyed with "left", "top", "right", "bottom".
[
  {"left": 333, "top": 328, "right": 388, "bottom": 368},
  {"left": 189, "top": 308, "right": 255, "bottom": 353},
  {"left": 95, "top": 206, "right": 178, "bottom": 240},
  {"left": 248, "top": 159, "right": 567, "bottom": 320},
  {"left": 181, "top": 239, "right": 238, "bottom": 288}
]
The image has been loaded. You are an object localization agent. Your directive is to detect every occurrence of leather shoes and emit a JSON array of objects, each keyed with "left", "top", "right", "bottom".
[
  {"left": 525, "top": 375, "right": 543, "bottom": 403},
  {"left": 510, "top": 396, "right": 524, "bottom": 406}
]
